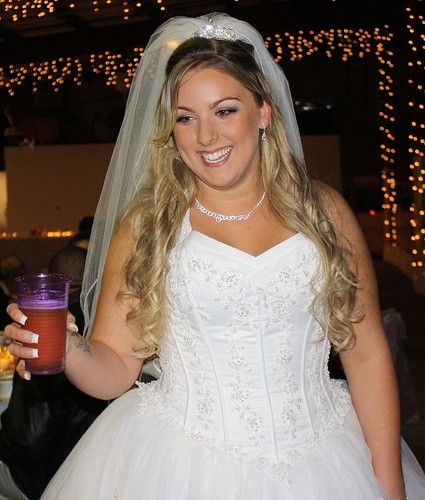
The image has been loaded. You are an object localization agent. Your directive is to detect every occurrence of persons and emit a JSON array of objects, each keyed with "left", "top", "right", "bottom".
[
  {"left": 48, "top": 232, "right": 91, "bottom": 281},
  {"left": 78, "top": 216, "right": 94, "bottom": 235},
  {"left": 4, "top": 22, "right": 425, "bottom": 499}
]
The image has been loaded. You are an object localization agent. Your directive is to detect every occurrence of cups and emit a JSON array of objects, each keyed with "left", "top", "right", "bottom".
[{"left": 15, "top": 274, "right": 73, "bottom": 375}]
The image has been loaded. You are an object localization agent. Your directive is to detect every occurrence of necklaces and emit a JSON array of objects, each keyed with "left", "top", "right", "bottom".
[{"left": 192, "top": 191, "right": 267, "bottom": 224}]
